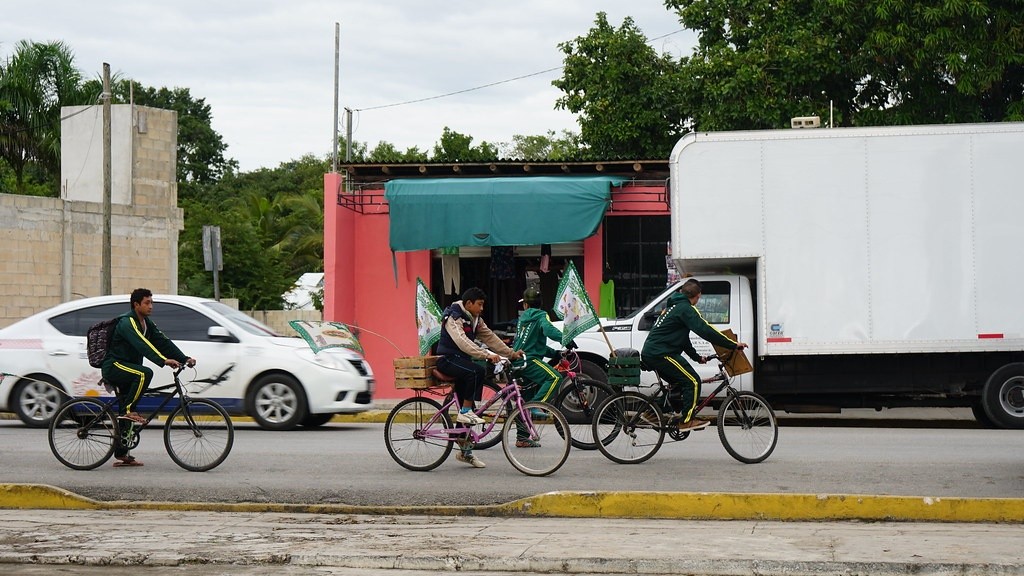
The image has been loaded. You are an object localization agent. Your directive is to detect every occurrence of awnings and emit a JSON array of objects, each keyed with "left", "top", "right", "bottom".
[{"left": 385, "top": 175, "right": 629, "bottom": 252}]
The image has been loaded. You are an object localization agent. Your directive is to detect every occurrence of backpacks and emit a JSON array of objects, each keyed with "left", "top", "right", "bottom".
[{"left": 86, "top": 315, "right": 130, "bottom": 368}]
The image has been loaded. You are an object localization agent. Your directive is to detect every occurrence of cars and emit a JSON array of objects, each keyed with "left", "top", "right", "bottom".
[
  {"left": 280, "top": 272, "right": 325, "bottom": 311},
  {"left": 0, "top": 294, "right": 376, "bottom": 429}
]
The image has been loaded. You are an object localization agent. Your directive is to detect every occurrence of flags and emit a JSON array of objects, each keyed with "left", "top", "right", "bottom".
[
  {"left": 552, "top": 264, "right": 600, "bottom": 348},
  {"left": 289, "top": 321, "right": 364, "bottom": 354},
  {"left": 415, "top": 277, "right": 444, "bottom": 358}
]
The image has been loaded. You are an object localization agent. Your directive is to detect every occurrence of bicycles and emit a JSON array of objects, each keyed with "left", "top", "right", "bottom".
[
  {"left": 384, "top": 353, "right": 571, "bottom": 477},
  {"left": 591, "top": 345, "right": 779, "bottom": 464},
  {"left": 47, "top": 361, "right": 235, "bottom": 472},
  {"left": 441, "top": 344, "right": 624, "bottom": 450}
]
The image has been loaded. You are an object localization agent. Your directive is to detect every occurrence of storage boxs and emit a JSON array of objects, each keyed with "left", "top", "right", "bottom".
[
  {"left": 609, "top": 356, "right": 641, "bottom": 385},
  {"left": 393, "top": 356, "right": 442, "bottom": 388}
]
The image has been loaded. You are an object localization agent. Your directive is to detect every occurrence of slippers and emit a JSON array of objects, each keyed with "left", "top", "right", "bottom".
[
  {"left": 113, "top": 456, "right": 144, "bottom": 466},
  {"left": 117, "top": 412, "right": 143, "bottom": 422}
]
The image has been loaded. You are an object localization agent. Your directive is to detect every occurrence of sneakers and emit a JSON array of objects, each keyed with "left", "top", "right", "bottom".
[
  {"left": 457, "top": 452, "right": 485, "bottom": 467},
  {"left": 640, "top": 410, "right": 659, "bottom": 427},
  {"left": 679, "top": 418, "right": 710, "bottom": 432},
  {"left": 515, "top": 439, "right": 541, "bottom": 447},
  {"left": 530, "top": 409, "right": 551, "bottom": 420},
  {"left": 457, "top": 409, "right": 486, "bottom": 424}
]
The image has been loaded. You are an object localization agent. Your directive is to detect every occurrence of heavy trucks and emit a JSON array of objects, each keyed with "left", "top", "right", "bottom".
[{"left": 494, "top": 122, "right": 1024, "bottom": 430}]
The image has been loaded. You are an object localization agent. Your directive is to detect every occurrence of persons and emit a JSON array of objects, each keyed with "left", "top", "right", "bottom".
[
  {"left": 436, "top": 288, "right": 526, "bottom": 468},
  {"left": 641, "top": 279, "right": 748, "bottom": 432},
  {"left": 507, "top": 288, "right": 578, "bottom": 448},
  {"left": 102, "top": 288, "right": 196, "bottom": 468}
]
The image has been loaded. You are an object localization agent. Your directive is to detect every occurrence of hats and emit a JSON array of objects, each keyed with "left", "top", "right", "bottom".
[{"left": 518, "top": 287, "right": 541, "bottom": 303}]
